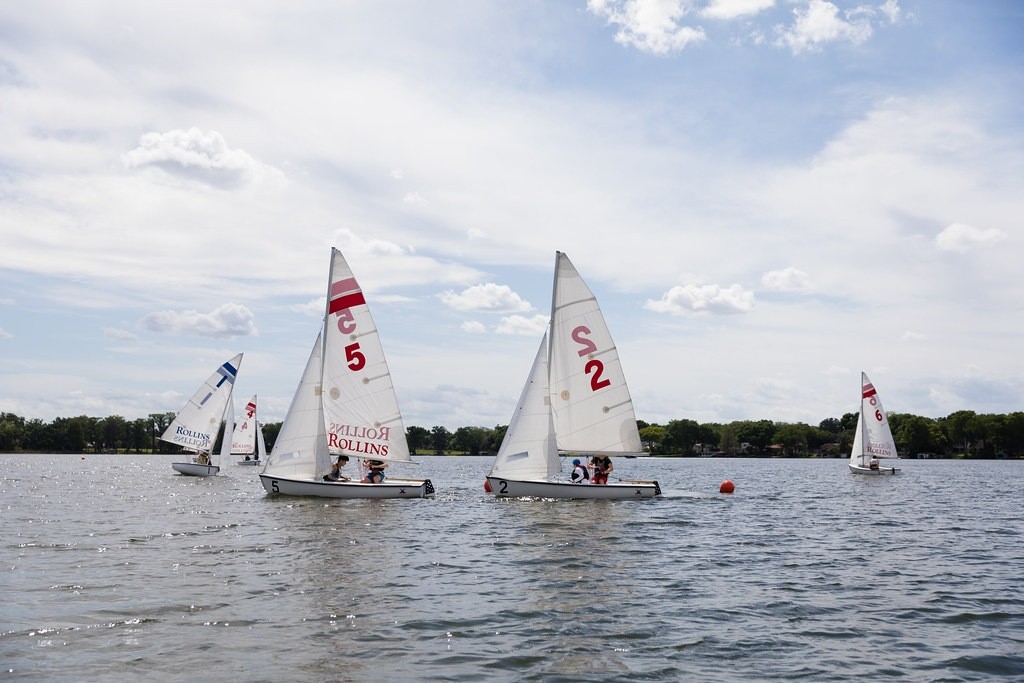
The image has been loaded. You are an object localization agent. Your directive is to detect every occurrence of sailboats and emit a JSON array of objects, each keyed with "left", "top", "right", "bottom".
[
  {"left": 485, "top": 250, "right": 662, "bottom": 499},
  {"left": 259, "top": 246, "right": 436, "bottom": 498},
  {"left": 155, "top": 352, "right": 243, "bottom": 476},
  {"left": 849, "top": 371, "right": 901, "bottom": 475},
  {"left": 230, "top": 395, "right": 267, "bottom": 466}
]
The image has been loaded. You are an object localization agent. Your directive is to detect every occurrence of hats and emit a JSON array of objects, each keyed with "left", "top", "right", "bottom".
[{"left": 573, "top": 459, "right": 580, "bottom": 464}]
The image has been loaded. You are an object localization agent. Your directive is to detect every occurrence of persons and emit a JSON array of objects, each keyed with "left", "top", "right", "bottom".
[
  {"left": 245, "top": 454, "right": 250, "bottom": 461},
  {"left": 197, "top": 450, "right": 209, "bottom": 464},
  {"left": 571, "top": 459, "right": 589, "bottom": 484},
  {"left": 870, "top": 455, "right": 879, "bottom": 469},
  {"left": 323, "top": 455, "right": 351, "bottom": 482},
  {"left": 588, "top": 455, "right": 613, "bottom": 484},
  {"left": 360, "top": 460, "right": 389, "bottom": 483}
]
